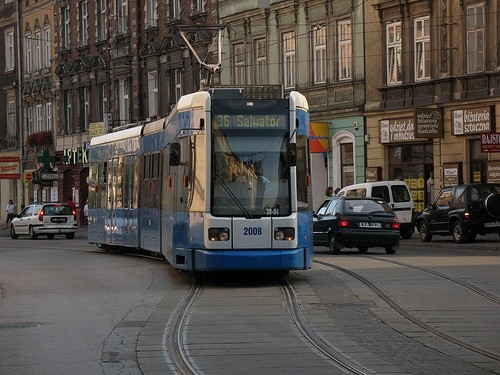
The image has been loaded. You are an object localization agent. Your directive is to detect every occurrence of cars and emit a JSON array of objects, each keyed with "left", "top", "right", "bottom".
[
  {"left": 313, "top": 195, "right": 400, "bottom": 254},
  {"left": 9, "top": 201, "right": 78, "bottom": 240}
]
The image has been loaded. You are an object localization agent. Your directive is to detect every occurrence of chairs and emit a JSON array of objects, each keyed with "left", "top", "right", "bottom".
[
  {"left": 362, "top": 203, "right": 382, "bottom": 212},
  {"left": 337, "top": 203, "right": 354, "bottom": 212}
]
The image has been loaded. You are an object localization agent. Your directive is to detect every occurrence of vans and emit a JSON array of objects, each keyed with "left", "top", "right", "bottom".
[{"left": 336, "top": 181, "right": 416, "bottom": 240}]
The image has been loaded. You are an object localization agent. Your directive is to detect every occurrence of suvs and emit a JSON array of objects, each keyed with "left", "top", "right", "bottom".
[{"left": 413, "top": 184, "right": 500, "bottom": 243}]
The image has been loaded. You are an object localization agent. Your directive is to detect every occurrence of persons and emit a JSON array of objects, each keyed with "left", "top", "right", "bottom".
[
  {"left": 334, "top": 187, "right": 341, "bottom": 194},
  {"left": 29, "top": 197, "right": 34, "bottom": 205},
  {"left": 3, "top": 200, "right": 16, "bottom": 228},
  {"left": 324, "top": 186, "right": 333, "bottom": 199},
  {"left": 68, "top": 195, "right": 77, "bottom": 213}
]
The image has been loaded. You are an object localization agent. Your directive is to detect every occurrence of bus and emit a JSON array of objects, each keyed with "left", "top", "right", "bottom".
[{"left": 86, "top": 86, "right": 313, "bottom": 285}]
